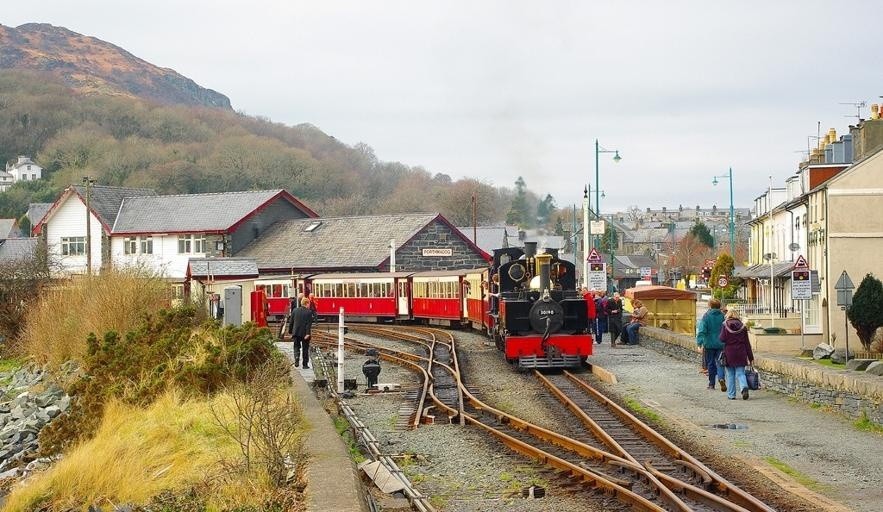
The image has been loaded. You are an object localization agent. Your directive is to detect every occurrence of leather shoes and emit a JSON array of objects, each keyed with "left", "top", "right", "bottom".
[{"left": 295, "top": 356, "right": 311, "bottom": 369}]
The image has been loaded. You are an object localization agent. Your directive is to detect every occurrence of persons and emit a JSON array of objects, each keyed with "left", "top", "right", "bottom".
[
  {"left": 575, "top": 287, "right": 648, "bottom": 346},
  {"left": 721, "top": 310, "right": 754, "bottom": 400},
  {"left": 286, "top": 297, "right": 312, "bottom": 368},
  {"left": 290, "top": 293, "right": 303, "bottom": 310},
  {"left": 259, "top": 285, "right": 267, "bottom": 313},
  {"left": 699, "top": 308, "right": 727, "bottom": 374},
  {"left": 489, "top": 274, "right": 500, "bottom": 301},
  {"left": 481, "top": 280, "right": 489, "bottom": 293},
  {"left": 209, "top": 294, "right": 224, "bottom": 318},
  {"left": 462, "top": 281, "right": 471, "bottom": 291},
  {"left": 308, "top": 294, "right": 319, "bottom": 326},
  {"left": 696, "top": 301, "right": 727, "bottom": 391}
]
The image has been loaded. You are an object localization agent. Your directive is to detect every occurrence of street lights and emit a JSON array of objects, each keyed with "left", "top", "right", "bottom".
[
  {"left": 710, "top": 166, "right": 735, "bottom": 258},
  {"left": 587, "top": 183, "right": 606, "bottom": 214},
  {"left": 591, "top": 135, "right": 622, "bottom": 263},
  {"left": 660, "top": 223, "right": 675, "bottom": 252}
]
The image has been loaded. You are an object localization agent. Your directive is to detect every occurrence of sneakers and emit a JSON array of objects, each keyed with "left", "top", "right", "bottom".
[
  {"left": 697, "top": 367, "right": 717, "bottom": 376},
  {"left": 706, "top": 380, "right": 749, "bottom": 400}
]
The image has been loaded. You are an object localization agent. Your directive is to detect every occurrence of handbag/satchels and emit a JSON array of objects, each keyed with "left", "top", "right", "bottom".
[
  {"left": 746, "top": 362, "right": 761, "bottom": 391},
  {"left": 720, "top": 350, "right": 728, "bottom": 368}
]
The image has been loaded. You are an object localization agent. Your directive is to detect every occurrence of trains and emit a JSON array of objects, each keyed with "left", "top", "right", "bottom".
[{"left": 42, "top": 241, "right": 594, "bottom": 373}]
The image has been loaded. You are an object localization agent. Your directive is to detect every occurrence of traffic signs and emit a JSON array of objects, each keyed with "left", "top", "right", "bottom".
[{"left": 718, "top": 277, "right": 727, "bottom": 288}]
